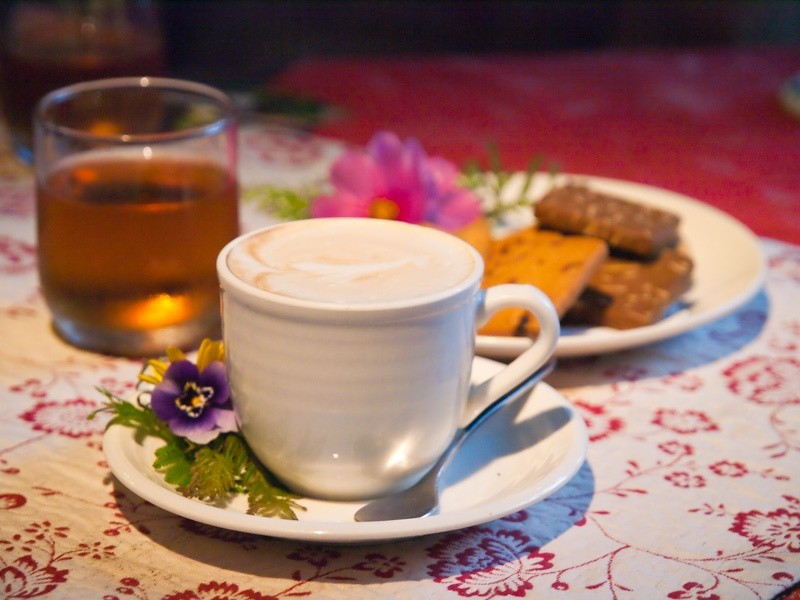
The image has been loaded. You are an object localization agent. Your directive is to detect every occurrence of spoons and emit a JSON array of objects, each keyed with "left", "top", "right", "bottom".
[{"left": 354, "top": 359, "right": 556, "bottom": 523}]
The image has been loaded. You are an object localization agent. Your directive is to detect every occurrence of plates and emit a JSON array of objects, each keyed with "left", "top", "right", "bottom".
[
  {"left": 101, "top": 353, "right": 590, "bottom": 543},
  {"left": 452, "top": 169, "right": 770, "bottom": 360}
]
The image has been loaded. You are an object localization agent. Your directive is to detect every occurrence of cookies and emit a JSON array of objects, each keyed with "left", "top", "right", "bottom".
[{"left": 453, "top": 184, "right": 694, "bottom": 339}]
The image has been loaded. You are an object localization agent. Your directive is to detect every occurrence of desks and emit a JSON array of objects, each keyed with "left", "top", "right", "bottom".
[{"left": 0, "top": 89, "right": 800, "bottom": 600}]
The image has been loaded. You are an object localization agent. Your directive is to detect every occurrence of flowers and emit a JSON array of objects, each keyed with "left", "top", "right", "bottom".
[
  {"left": 242, "top": 130, "right": 563, "bottom": 229},
  {"left": 86, "top": 337, "right": 308, "bottom": 521}
]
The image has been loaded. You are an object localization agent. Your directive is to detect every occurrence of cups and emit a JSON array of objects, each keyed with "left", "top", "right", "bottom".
[
  {"left": 217, "top": 216, "right": 560, "bottom": 500},
  {"left": 31, "top": 77, "right": 244, "bottom": 359}
]
